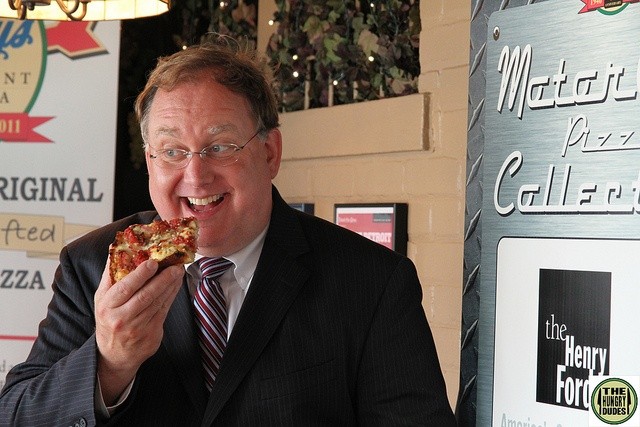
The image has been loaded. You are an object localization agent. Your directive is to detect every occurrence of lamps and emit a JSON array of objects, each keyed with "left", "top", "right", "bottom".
[{"left": 0, "top": 0, "right": 172, "bottom": 22}]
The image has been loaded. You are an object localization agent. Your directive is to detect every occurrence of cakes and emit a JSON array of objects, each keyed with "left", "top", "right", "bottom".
[{"left": 108, "top": 216, "right": 198, "bottom": 286}]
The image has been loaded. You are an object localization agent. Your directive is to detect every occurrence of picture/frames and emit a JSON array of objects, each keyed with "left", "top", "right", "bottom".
[
  {"left": 334, "top": 202, "right": 408, "bottom": 256},
  {"left": 288, "top": 203, "right": 314, "bottom": 216}
]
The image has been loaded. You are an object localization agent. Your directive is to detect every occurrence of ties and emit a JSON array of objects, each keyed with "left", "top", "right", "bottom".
[{"left": 190, "top": 257, "right": 233, "bottom": 401}]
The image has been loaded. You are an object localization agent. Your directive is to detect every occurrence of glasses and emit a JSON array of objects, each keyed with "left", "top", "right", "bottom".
[{"left": 146, "top": 125, "right": 267, "bottom": 165}]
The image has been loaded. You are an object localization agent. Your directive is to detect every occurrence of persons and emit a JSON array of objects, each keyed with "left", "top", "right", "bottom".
[{"left": 1, "top": 43, "right": 456, "bottom": 427}]
